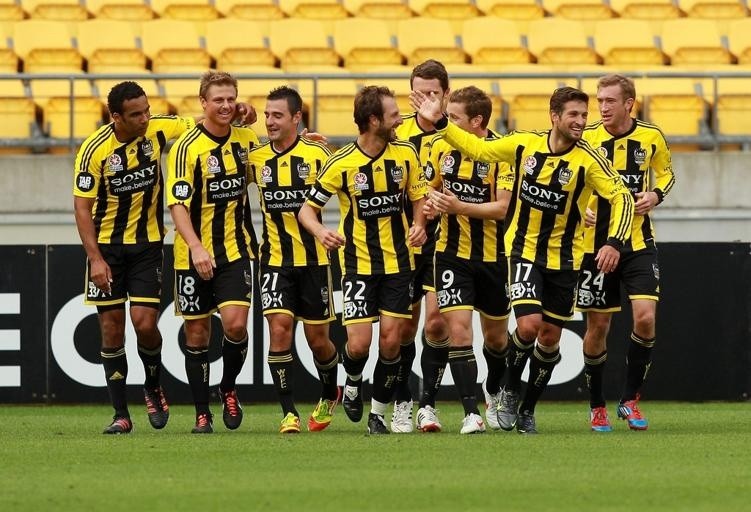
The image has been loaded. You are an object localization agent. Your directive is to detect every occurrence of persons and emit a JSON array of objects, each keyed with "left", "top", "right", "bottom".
[
  {"left": 574, "top": 74, "right": 677, "bottom": 433},
  {"left": 422, "top": 84, "right": 515, "bottom": 436},
  {"left": 297, "top": 83, "right": 433, "bottom": 437},
  {"left": 249, "top": 86, "right": 343, "bottom": 437},
  {"left": 392, "top": 59, "right": 453, "bottom": 436},
  {"left": 165, "top": 69, "right": 330, "bottom": 439},
  {"left": 72, "top": 80, "right": 260, "bottom": 437},
  {"left": 403, "top": 86, "right": 638, "bottom": 436}
]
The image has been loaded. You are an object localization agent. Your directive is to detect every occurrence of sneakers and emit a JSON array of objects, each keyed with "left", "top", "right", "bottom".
[
  {"left": 590, "top": 406, "right": 613, "bottom": 433},
  {"left": 218, "top": 385, "right": 242, "bottom": 430},
  {"left": 104, "top": 413, "right": 131, "bottom": 433},
  {"left": 143, "top": 384, "right": 169, "bottom": 429},
  {"left": 279, "top": 377, "right": 538, "bottom": 435},
  {"left": 617, "top": 393, "right": 648, "bottom": 430},
  {"left": 191, "top": 410, "right": 213, "bottom": 433}
]
